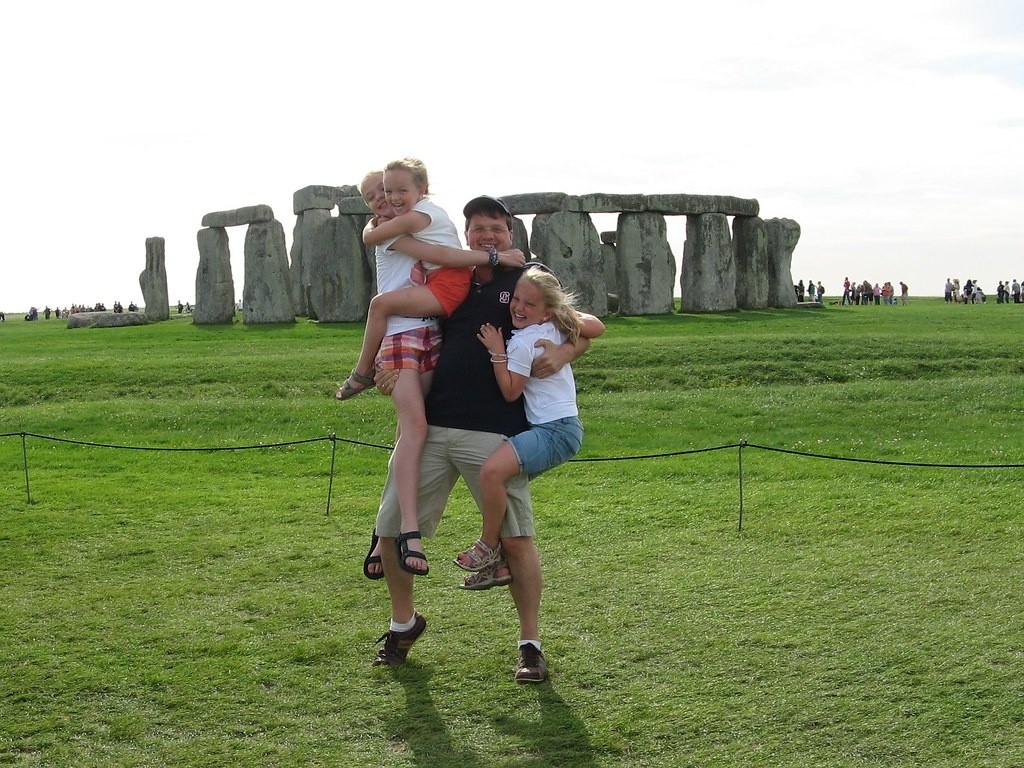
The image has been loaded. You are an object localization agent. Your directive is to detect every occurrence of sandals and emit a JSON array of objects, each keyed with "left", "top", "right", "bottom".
[
  {"left": 394, "top": 530, "right": 429, "bottom": 575},
  {"left": 364, "top": 527, "right": 386, "bottom": 580},
  {"left": 453, "top": 540, "right": 502, "bottom": 572},
  {"left": 458, "top": 561, "right": 512, "bottom": 590},
  {"left": 335, "top": 369, "right": 376, "bottom": 401}
]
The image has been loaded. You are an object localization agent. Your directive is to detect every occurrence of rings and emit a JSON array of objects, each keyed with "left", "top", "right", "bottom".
[{"left": 382, "top": 384, "right": 386, "bottom": 388}]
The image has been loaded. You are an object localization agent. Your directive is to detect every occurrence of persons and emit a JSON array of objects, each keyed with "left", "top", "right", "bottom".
[
  {"left": 1012, "top": 279, "right": 1020, "bottom": 303},
  {"left": 560, "top": 311, "right": 605, "bottom": 339},
  {"left": 808, "top": 280, "right": 815, "bottom": 302},
  {"left": 998, "top": 281, "right": 1004, "bottom": 303},
  {"left": 900, "top": 282, "right": 909, "bottom": 306},
  {"left": 882, "top": 282, "right": 894, "bottom": 304},
  {"left": 842, "top": 277, "right": 850, "bottom": 305},
  {"left": 1021, "top": 281, "right": 1024, "bottom": 302},
  {"left": 453, "top": 266, "right": 585, "bottom": 590},
  {"left": 851, "top": 280, "right": 873, "bottom": 306},
  {"left": 817, "top": 281, "right": 822, "bottom": 302},
  {"left": 945, "top": 279, "right": 953, "bottom": 302},
  {"left": 953, "top": 279, "right": 960, "bottom": 301},
  {"left": 335, "top": 157, "right": 473, "bottom": 401},
  {"left": 798, "top": 279, "right": 804, "bottom": 296},
  {"left": 357, "top": 169, "right": 526, "bottom": 580},
  {"left": 874, "top": 283, "right": 881, "bottom": 305},
  {"left": 964, "top": 279, "right": 974, "bottom": 304},
  {"left": 372, "top": 196, "right": 590, "bottom": 682},
  {"left": 972, "top": 280, "right": 977, "bottom": 304},
  {"left": 1005, "top": 281, "right": 1009, "bottom": 303}
]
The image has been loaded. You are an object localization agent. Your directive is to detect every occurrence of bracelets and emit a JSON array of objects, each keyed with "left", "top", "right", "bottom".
[
  {"left": 489, "top": 350, "right": 507, "bottom": 363},
  {"left": 487, "top": 246, "right": 499, "bottom": 267}
]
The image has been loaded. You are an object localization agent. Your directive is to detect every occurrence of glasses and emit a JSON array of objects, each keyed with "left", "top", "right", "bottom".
[{"left": 467, "top": 227, "right": 508, "bottom": 236}]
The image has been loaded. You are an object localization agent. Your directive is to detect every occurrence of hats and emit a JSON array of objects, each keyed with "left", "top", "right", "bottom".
[{"left": 463, "top": 195, "right": 511, "bottom": 217}]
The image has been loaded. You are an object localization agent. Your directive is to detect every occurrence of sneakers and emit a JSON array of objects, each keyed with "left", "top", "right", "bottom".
[
  {"left": 371, "top": 609, "right": 428, "bottom": 666},
  {"left": 515, "top": 642, "right": 547, "bottom": 681}
]
